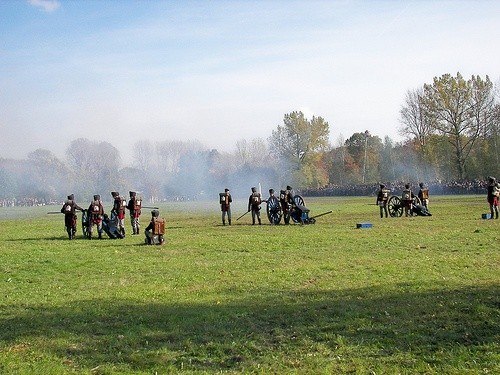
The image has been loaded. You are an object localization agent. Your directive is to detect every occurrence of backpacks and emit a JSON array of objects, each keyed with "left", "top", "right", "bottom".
[
  {"left": 252, "top": 192, "right": 261, "bottom": 206},
  {"left": 402, "top": 190, "right": 411, "bottom": 200},
  {"left": 133, "top": 199, "right": 141, "bottom": 210},
  {"left": 421, "top": 189, "right": 428, "bottom": 200},
  {"left": 280, "top": 190, "right": 289, "bottom": 203},
  {"left": 491, "top": 183, "right": 500, "bottom": 197},
  {"left": 65, "top": 200, "right": 75, "bottom": 215},
  {"left": 116, "top": 198, "right": 126, "bottom": 210},
  {"left": 382, "top": 189, "right": 389, "bottom": 201},
  {"left": 91, "top": 201, "right": 102, "bottom": 217},
  {"left": 152, "top": 217, "right": 165, "bottom": 235},
  {"left": 219, "top": 193, "right": 228, "bottom": 204}
]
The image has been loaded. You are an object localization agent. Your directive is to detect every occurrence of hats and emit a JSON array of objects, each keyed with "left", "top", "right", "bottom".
[
  {"left": 287, "top": 186, "right": 292, "bottom": 190},
  {"left": 489, "top": 176, "right": 495, "bottom": 181},
  {"left": 419, "top": 183, "right": 424, "bottom": 187},
  {"left": 129, "top": 191, "right": 136, "bottom": 197},
  {"left": 269, "top": 189, "right": 275, "bottom": 193},
  {"left": 111, "top": 192, "right": 119, "bottom": 197},
  {"left": 251, "top": 187, "right": 257, "bottom": 191},
  {"left": 380, "top": 184, "right": 385, "bottom": 187},
  {"left": 405, "top": 184, "right": 410, "bottom": 188},
  {"left": 94, "top": 194, "right": 100, "bottom": 200},
  {"left": 152, "top": 210, "right": 159, "bottom": 217},
  {"left": 67, "top": 194, "right": 74, "bottom": 200}
]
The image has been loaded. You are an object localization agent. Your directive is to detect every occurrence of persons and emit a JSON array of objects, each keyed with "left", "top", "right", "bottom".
[
  {"left": 110, "top": 192, "right": 126, "bottom": 236},
  {"left": 376, "top": 184, "right": 388, "bottom": 218},
  {"left": 85, "top": 194, "right": 105, "bottom": 240},
  {"left": 268, "top": 189, "right": 279, "bottom": 203},
  {"left": 61, "top": 194, "right": 85, "bottom": 240},
  {"left": 144, "top": 209, "right": 166, "bottom": 245},
  {"left": 486, "top": 176, "right": 499, "bottom": 219},
  {"left": 403, "top": 182, "right": 414, "bottom": 217},
  {"left": 285, "top": 186, "right": 295, "bottom": 218},
  {"left": 125, "top": 191, "right": 140, "bottom": 235},
  {"left": 419, "top": 182, "right": 429, "bottom": 212},
  {"left": 248, "top": 186, "right": 263, "bottom": 226},
  {"left": 220, "top": 189, "right": 232, "bottom": 226}
]
